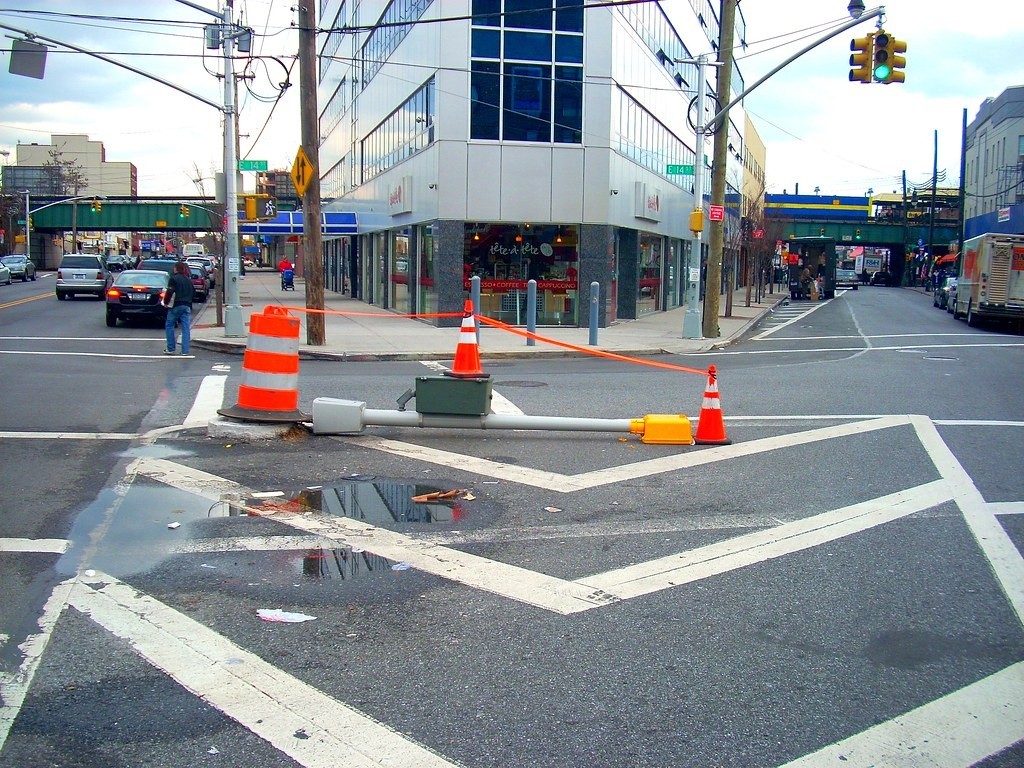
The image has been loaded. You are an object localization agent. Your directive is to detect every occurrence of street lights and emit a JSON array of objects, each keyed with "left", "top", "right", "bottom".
[{"left": 703, "top": 4, "right": 866, "bottom": 340}]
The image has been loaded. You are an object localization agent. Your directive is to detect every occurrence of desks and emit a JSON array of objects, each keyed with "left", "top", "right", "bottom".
[{"left": 479, "top": 291, "right": 510, "bottom": 321}]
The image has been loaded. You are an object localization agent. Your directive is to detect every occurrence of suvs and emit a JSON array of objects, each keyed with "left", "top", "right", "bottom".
[
  {"left": 186, "top": 257, "right": 217, "bottom": 289},
  {"left": 55, "top": 250, "right": 115, "bottom": 301}
]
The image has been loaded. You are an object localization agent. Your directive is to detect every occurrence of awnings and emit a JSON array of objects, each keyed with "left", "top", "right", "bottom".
[
  {"left": 137, "top": 231, "right": 165, "bottom": 235},
  {"left": 937, "top": 253, "right": 958, "bottom": 264}
]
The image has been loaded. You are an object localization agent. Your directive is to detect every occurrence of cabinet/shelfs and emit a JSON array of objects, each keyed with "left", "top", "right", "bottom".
[{"left": 550, "top": 289, "right": 569, "bottom": 326}]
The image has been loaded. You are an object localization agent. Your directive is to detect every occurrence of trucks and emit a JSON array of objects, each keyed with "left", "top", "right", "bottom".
[
  {"left": 953, "top": 231, "right": 1024, "bottom": 330},
  {"left": 787, "top": 236, "right": 837, "bottom": 298}
]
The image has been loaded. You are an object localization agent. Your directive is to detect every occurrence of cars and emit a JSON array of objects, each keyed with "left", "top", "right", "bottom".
[
  {"left": 105, "top": 269, "right": 181, "bottom": 328},
  {"left": 136, "top": 260, "right": 192, "bottom": 281},
  {"left": 0, "top": 254, "right": 36, "bottom": 285},
  {"left": 244, "top": 257, "right": 254, "bottom": 267},
  {"left": 106, "top": 254, "right": 138, "bottom": 272},
  {"left": 835, "top": 270, "right": 860, "bottom": 290},
  {"left": 933, "top": 277, "right": 958, "bottom": 314},
  {"left": 870, "top": 271, "right": 891, "bottom": 286},
  {"left": 206, "top": 254, "right": 220, "bottom": 270},
  {"left": 184, "top": 262, "right": 211, "bottom": 303}
]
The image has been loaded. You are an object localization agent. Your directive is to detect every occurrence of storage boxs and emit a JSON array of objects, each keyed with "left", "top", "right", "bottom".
[{"left": 811, "top": 283, "right": 818, "bottom": 301}]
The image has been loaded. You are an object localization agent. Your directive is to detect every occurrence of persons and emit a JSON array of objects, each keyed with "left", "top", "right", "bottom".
[
  {"left": 154, "top": 253, "right": 181, "bottom": 260},
  {"left": 163, "top": 261, "right": 197, "bottom": 356},
  {"left": 924, "top": 266, "right": 958, "bottom": 292},
  {"left": 800, "top": 266, "right": 814, "bottom": 300},
  {"left": 278, "top": 255, "right": 295, "bottom": 291},
  {"left": 122, "top": 254, "right": 141, "bottom": 271},
  {"left": 253, "top": 256, "right": 263, "bottom": 268},
  {"left": 876, "top": 207, "right": 892, "bottom": 223},
  {"left": 210, "top": 258, "right": 215, "bottom": 272}
]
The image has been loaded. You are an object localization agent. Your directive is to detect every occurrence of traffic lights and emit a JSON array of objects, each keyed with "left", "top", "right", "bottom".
[
  {"left": 30, "top": 218, "right": 33, "bottom": 227},
  {"left": 186, "top": 208, "right": 189, "bottom": 217},
  {"left": 92, "top": 202, "right": 96, "bottom": 212},
  {"left": 98, "top": 202, "right": 102, "bottom": 212},
  {"left": 848, "top": 30, "right": 907, "bottom": 85},
  {"left": 180, "top": 208, "right": 183, "bottom": 218},
  {"left": 254, "top": 196, "right": 278, "bottom": 218}
]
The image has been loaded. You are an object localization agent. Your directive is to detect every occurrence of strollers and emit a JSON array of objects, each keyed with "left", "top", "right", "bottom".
[{"left": 281, "top": 269, "right": 295, "bottom": 291}]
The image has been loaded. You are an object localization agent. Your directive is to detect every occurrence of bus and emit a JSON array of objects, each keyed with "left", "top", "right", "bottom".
[{"left": 182, "top": 243, "right": 204, "bottom": 258}]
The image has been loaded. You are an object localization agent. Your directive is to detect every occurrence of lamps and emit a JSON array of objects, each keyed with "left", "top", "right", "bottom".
[
  {"left": 473, "top": 223, "right": 479, "bottom": 240},
  {"left": 515, "top": 225, "right": 522, "bottom": 241},
  {"left": 557, "top": 225, "right": 562, "bottom": 242}
]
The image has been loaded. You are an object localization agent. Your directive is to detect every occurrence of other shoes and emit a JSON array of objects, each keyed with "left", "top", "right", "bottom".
[
  {"left": 163, "top": 349, "right": 173, "bottom": 354},
  {"left": 181, "top": 351, "right": 187, "bottom": 355}
]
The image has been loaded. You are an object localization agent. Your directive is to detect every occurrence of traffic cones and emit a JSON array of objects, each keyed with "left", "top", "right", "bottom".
[
  {"left": 443, "top": 294, "right": 492, "bottom": 380},
  {"left": 693, "top": 364, "right": 733, "bottom": 446}
]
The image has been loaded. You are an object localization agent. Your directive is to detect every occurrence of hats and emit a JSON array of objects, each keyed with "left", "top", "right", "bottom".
[{"left": 808, "top": 265, "right": 813, "bottom": 271}]
